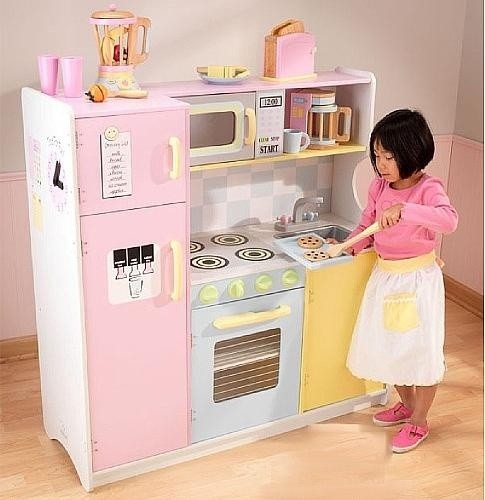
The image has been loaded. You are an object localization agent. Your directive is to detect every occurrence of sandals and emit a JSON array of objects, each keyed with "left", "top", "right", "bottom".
[
  {"left": 391, "top": 419, "right": 431, "bottom": 454},
  {"left": 371, "top": 401, "right": 415, "bottom": 427}
]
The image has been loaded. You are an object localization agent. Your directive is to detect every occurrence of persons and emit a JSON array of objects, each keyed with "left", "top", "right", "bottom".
[{"left": 323, "top": 108, "right": 458, "bottom": 453}]
